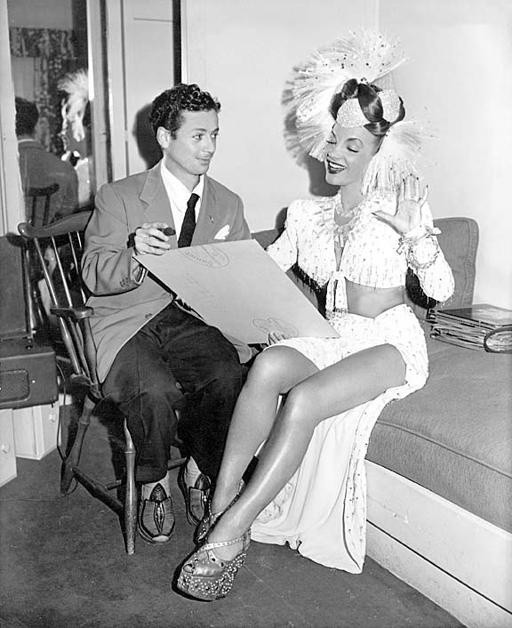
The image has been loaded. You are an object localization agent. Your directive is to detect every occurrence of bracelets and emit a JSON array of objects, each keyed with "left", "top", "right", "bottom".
[{"left": 401, "top": 226, "right": 439, "bottom": 270}]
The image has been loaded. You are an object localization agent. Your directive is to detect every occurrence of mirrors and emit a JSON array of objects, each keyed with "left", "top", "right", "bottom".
[{"left": 2, "top": 0, "right": 108, "bottom": 344}]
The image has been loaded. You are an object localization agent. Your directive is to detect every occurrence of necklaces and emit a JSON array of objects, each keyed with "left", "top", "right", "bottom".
[{"left": 334, "top": 192, "right": 361, "bottom": 219}]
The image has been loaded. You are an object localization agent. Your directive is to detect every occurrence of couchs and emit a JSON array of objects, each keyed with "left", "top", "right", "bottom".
[{"left": 245, "top": 218, "right": 512, "bottom": 628}]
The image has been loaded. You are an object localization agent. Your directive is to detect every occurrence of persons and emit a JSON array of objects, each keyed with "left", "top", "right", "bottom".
[
  {"left": 80, "top": 84, "right": 249, "bottom": 545},
  {"left": 177, "top": 79, "right": 454, "bottom": 601},
  {"left": 16, "top": 97, "right": 95, "bottom": 330}
]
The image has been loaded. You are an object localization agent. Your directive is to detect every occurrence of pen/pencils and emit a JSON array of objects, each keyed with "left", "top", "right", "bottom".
[{"left": 164, "top": 227, "right": 174, "bottom": 236}]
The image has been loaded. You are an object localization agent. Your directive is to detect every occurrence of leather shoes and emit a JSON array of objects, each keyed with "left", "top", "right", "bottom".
[
  {"left": 178, "top": 462, "right": 210, "bottom": 526},
  {"left": 138, "top": 471, "right": 175, "bottom": 544}
]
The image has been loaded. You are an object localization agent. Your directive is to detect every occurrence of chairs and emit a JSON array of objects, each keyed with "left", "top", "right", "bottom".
[
  {"left": 19, "top": 210, "right": 191, "bottom": 557},
  {"left": 21, "top": 182, "right": 63, "bottom": 337}
]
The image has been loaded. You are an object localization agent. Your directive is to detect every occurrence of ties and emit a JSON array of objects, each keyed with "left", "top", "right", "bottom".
[{"left": 177, "top": 192, "right": 200, "bottom": 248}]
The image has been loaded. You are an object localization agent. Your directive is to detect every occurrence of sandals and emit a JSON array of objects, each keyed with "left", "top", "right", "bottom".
[{"left": 177, "top": 478, "right": 252, "bottom": 601}]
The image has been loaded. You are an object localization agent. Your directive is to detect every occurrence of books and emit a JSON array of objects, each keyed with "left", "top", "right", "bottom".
[{"left": 430, "top": 307, "right": 512, "bottom": 353}]
